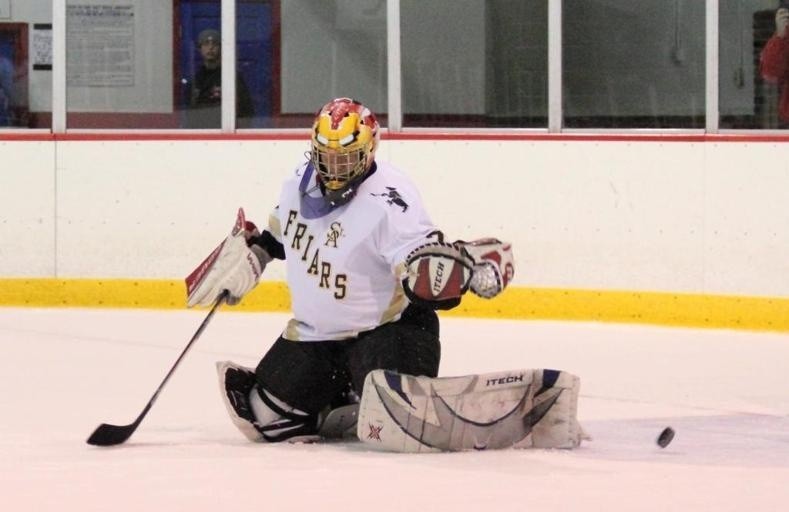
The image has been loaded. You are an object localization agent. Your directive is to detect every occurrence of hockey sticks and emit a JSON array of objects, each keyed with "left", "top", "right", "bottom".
[{"left": 76, "top": 291, "right": 228, "bottom": 446}]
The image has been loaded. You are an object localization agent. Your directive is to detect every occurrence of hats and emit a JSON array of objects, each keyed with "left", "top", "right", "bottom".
[{"left": 197, "top": 29, "right": 220, "bottom": 44}]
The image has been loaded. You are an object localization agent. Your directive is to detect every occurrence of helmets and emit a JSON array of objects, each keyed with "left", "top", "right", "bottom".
[{"left": 303, "top": 97, "right": 380, "bottom": 191}]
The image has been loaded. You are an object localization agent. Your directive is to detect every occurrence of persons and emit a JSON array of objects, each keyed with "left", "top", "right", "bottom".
[
  {"left": 186, "top": 97, "right": 581, "bottom": 453},
  {"left": 760, "top": 1, "right": 788, "bottom": 128},
  {"left": 179, "top": 26, "right": 252, "bottom": 132}
]
